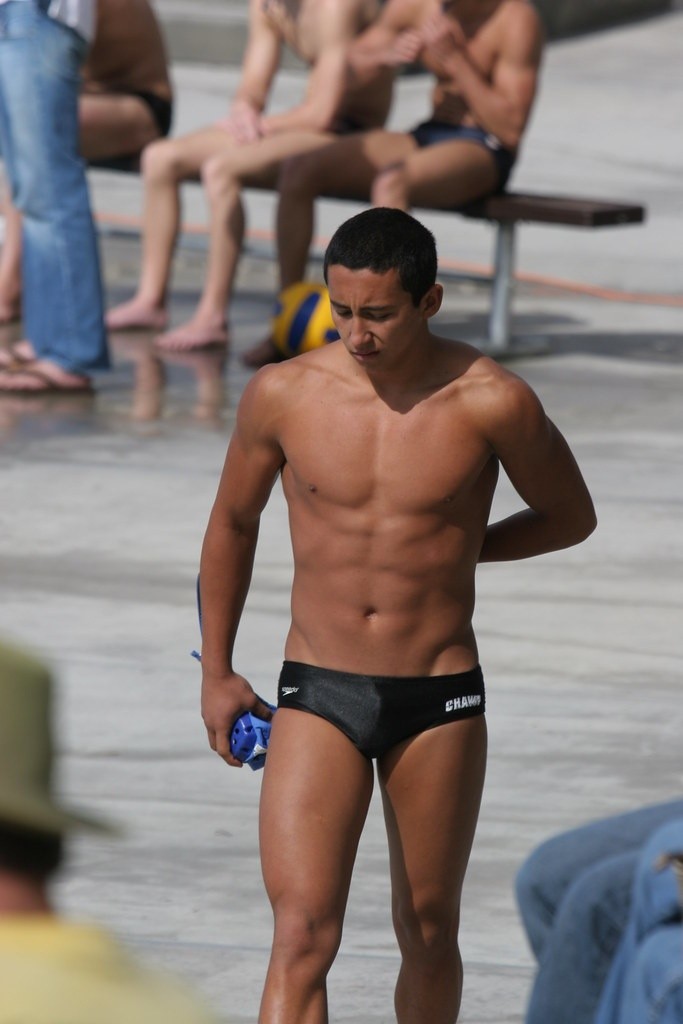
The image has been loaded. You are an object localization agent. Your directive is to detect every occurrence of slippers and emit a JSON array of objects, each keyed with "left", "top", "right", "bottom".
[
  {"left": 0, "top": 342, "right": 41, "bottom": 367},
  {"left": 0, "top": 359, "right": 95, "bottom": 395}
]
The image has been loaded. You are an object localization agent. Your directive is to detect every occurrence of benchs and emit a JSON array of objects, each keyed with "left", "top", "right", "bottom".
[{"left": 88, "top": 148, "right": 647, "bottom": 356}]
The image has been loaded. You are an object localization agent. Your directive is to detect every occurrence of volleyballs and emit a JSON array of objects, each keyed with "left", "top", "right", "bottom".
[{"left": 270, "top": 280, "right": 341, "bottom": 357}]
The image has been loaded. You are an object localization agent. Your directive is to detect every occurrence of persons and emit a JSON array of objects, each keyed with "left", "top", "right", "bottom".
[
  {"left": 193, "top": 202, "right": 600, "bottom": 1024},
  {"left": 1, "top": 641, "right": 222, "bottom": 1021},
  {"left": 0, "top": 1, "right": 550, "bottom": 396},
  {"left": 511, "top": 798, "right": 682, "bottom": 1023}
]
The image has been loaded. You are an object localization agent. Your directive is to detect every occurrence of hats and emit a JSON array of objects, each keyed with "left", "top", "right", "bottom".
[{"left": 0, "top": 645, "right": 125, "bottom": 839}]
